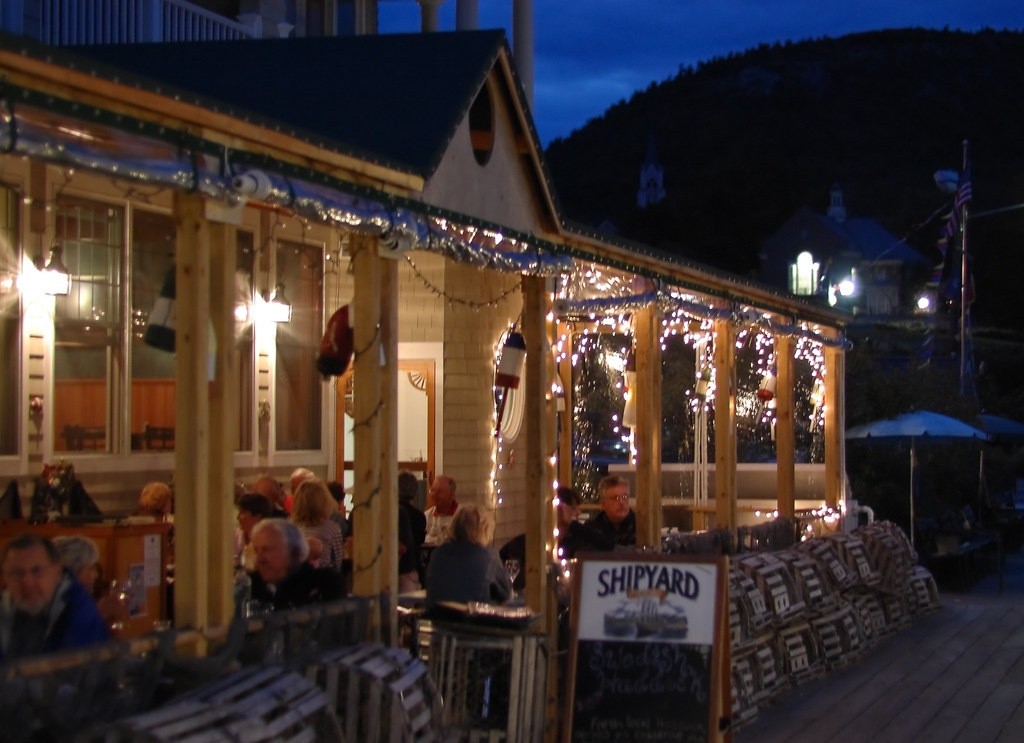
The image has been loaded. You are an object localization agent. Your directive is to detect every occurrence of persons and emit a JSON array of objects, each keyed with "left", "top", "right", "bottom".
[
  {"left": 427, "top": 506, "right": 510, "bottom": 604},
  {"left": 424, "top": 474, "right": 462, "bottom": 546},
  {"left": 499, "top": 486, "right": 615, "bottom": 591},
  {"left": 398, "top": 473, "right": 426, "bottom": 595},
  {"left": 0, "top": 535, "right": 113, "bottom": 660},
  {"left": 583, "top": 474, "right": 637, "bottom": 546},
  {"left": 140, "top": 481, "right": 171, "bottom": 516},
  {"left": 234, "top": 467, "right": 353, "bottom": 616},
  {"left": 250, "top": 518, "right": 331, "bottom": 610}
]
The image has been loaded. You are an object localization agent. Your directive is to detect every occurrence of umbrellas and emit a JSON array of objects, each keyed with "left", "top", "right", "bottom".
[
  {"left": 956, "top": 413, "right": 1024, "bottom": 525},
  {"left": 844, "top": 409, "right": 991, "bottom": 545}
]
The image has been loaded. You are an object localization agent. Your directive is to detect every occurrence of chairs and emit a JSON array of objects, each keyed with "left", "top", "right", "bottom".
[{"left": 661, "top": 503, "right": 692, "bottom": 534}]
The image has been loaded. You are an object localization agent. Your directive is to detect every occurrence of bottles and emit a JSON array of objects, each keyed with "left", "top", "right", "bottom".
[
  {"left": 234, "top": 565, "right": 252, "bottom": 617},
  {"left": 127, "top": 562, "right": 148, "bottom": 617},
  {"left": 427, "top": 516, "right": 443, "bottom": 545}
]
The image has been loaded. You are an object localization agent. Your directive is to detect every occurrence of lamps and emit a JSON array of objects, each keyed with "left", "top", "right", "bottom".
[
  {"left": 262, "top": 264, "right": 294, "bottom": 324},
  {"left": 32, "top": 224, "right": 72, "bottom": 298},
  {"left": 222, "top": 144, "right": 271, "bottom": 204}
]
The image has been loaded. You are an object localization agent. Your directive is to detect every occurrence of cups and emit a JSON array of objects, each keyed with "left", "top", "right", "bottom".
[{"left": 636, "top": 545, "right": 653, "bottom": 561}]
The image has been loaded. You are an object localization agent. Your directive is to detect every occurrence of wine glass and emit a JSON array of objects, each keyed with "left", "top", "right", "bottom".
[{"left": 504, "top": 560, "right": 520, "bottom": 600}]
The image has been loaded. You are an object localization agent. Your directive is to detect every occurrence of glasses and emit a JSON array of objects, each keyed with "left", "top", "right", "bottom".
[
  {"left": 602, "top": 492, "right": 630, "bottom": 502},
  {"left": 429, "top": 486, "right": 449, "bottom": 495}
]
